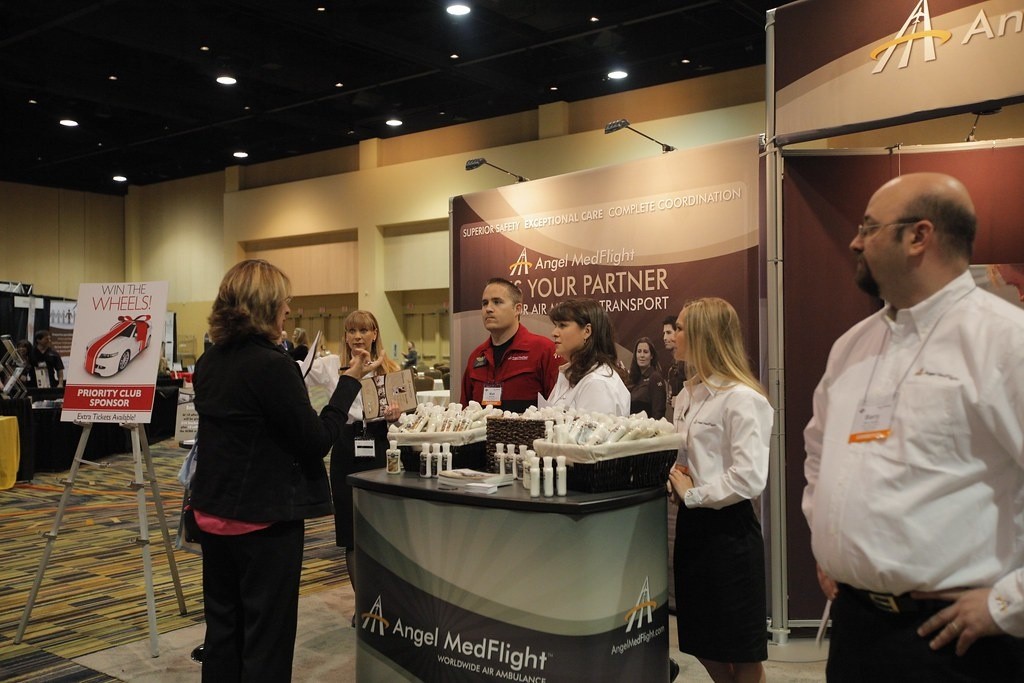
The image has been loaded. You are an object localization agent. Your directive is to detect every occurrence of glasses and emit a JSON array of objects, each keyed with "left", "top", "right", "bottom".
[{"left": 857, "top": 220, "right": 921, "bottom": 243}]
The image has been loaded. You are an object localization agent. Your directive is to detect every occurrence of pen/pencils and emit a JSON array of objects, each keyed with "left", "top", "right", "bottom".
[{"left": 340, "top": 362, "right": 373, "bottom": 371}]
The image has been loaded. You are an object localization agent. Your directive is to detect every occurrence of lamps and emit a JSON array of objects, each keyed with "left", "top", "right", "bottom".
[
  {"left": 965, "top": 107, "right": 1003, "bottom": 142},
  {"left": 604, "top": 118, "right": 675, "bottom": 151},
  {"left": 465, "top": 158, "right": 530, "bottom": 184}
]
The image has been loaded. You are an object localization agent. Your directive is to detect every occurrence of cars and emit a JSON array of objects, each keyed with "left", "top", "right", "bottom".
[{"left": 85, "top": 318, "right": 153, "bottom": 377}]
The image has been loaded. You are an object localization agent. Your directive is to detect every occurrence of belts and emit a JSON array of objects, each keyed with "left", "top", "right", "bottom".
[{"left": 832, "top": 578, "right": 956, "bottom": 614}]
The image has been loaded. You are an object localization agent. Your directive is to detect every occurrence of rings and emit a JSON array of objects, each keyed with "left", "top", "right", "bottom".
[
  {"left": 389, "top": 412, "right": 392, "bottom": 416},
  {"left": 952, "top": 622, "right": 958, "bottom": 631}
]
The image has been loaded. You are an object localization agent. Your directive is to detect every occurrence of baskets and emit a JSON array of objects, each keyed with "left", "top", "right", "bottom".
[
  {"left": 388, "top": 424, "right": 490, "bottom": 476},
  {"left": 529, "top": 433, "right": 682, "bottom": 493},
  {"left": 484, "top": 416, "right": 558, "bottom": 475}
]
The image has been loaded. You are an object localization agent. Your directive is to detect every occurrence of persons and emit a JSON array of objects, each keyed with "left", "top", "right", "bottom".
[
  {"left": 279, "top": 327, "right": 309, "bottom": 360},
  {"left": 626, "top": 337, "right": 667, "bottom": 419},
  {"left": 180, "top": 259, "right": 386, "bottom": 683},
  {"left": 329, "top": 310, "right": 402, "bottom": 628},
  {"left": 460, "top": 278, "right": 568, "bottom": 413},
  {"left": 667, "top": 297, "right": 775, "bottom": 683},
  {"left": 546, "top": 299, "right": 679, "bottom": 683},
  {"left": 0, "top": 330, "right": 64, "bottom": 387},
  {"left": 802, "top": 173, "right": 1024, "bottom": 683},
  {"left": 663, "top": 315, "right": 688, "bottom": 407},
  {"left": 402, "top": 341, "right": 416, "bottom": 368}
]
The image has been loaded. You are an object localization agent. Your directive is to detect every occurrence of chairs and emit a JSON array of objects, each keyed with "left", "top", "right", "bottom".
[{"left": 408, "top": 360, "right": 451, "bottom": 393}]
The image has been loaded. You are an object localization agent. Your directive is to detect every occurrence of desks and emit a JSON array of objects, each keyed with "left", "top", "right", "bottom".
[
  {"left": 32, "top": 385, "right": 179, "bottom": 474},
  {"left": 344, "top": 468, "right": 672, "bottom": 683}
]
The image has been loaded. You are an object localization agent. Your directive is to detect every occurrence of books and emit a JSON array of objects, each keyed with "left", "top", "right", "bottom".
[{"left": 360, "top": 369, "right": 417, "bottom": 420}]
